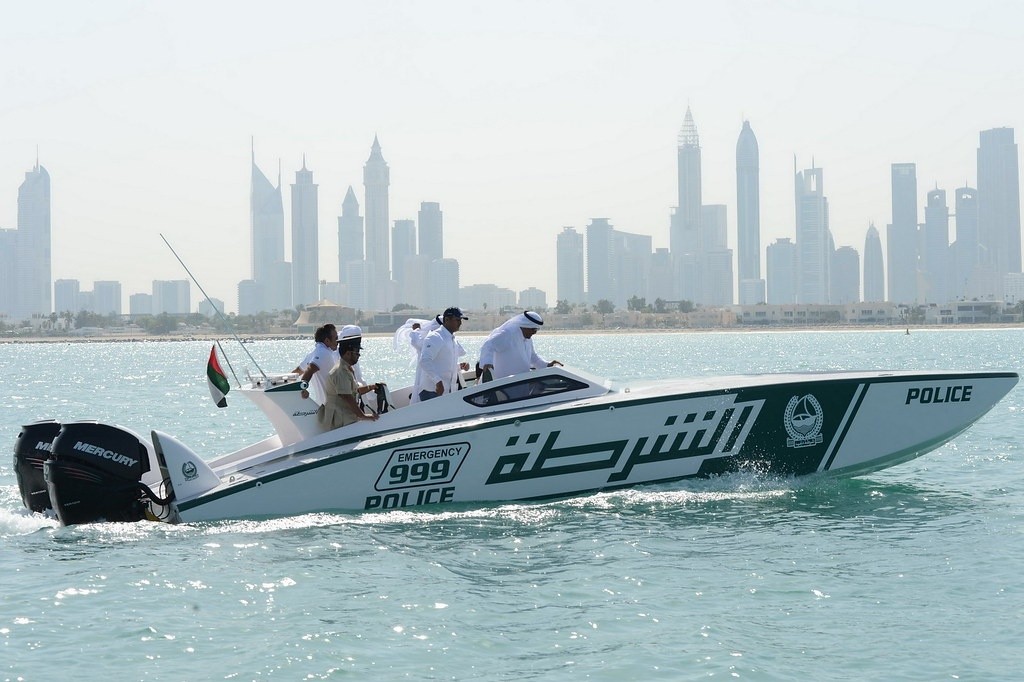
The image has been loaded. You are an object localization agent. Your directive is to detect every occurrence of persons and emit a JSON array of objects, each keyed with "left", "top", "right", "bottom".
[
  {"left": 292, "top": 323, "right": 338, "bottom": 405},
  {"left": 408, "top": 307, "right": 469, "bottom": 405},
  {"left": 479, "top": 312, "right": 563, "bottom": 402},
  {"left": 333, "top": 325, "right": 367, "bottom": 386},
  {"left": 317, "top": 336, "right": 377, "bottom": 431}
]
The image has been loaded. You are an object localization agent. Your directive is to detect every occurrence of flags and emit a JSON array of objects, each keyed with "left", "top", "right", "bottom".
[{"left": 206, "top": 345, "right": 230, "bottom": 408}]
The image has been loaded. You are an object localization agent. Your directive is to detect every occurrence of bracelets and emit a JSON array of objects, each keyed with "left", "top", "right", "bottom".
[{"left": 368, "top": 385, "right": 370, "bottom": 390}]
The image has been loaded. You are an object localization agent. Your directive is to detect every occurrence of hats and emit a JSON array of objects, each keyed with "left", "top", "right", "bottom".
[
  {"left": 335, "top": 333, "right": 363, "bottom": 349},
  {"left": 444, "top": 306, "right": 469, "bottom": 320}
]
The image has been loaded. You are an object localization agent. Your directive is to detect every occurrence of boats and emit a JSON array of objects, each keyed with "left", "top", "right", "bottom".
[{"left": 8, "top": 233, "right": 1020, "bottom": 535}]
